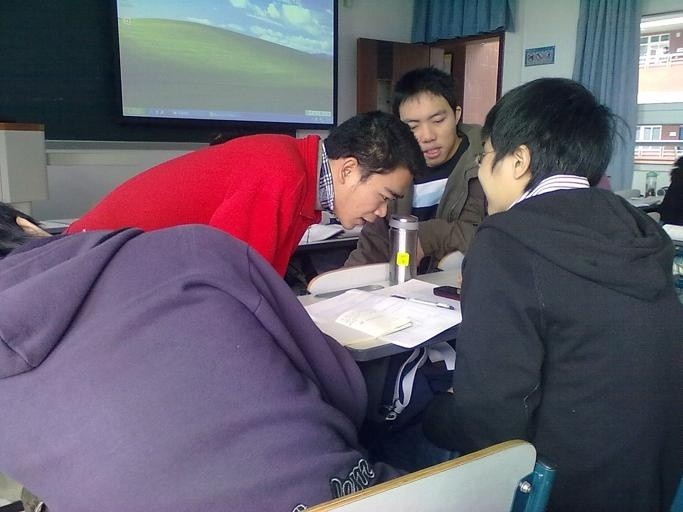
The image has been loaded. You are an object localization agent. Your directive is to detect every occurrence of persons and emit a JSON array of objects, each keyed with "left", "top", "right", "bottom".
[
  {"left": 0, "top": 200, "right": 414, "bottom": 511},
  {"left": 341, "top": 64, "right": 488, "bottom": 276},
  {"left": 57, "top": 108, "right": 427, "bottom": 282},
  {"left": 357, "top": 77, "right": 682, "bottom": 510}
]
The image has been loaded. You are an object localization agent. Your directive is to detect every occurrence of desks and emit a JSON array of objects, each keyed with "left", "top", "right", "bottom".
[{"left": 37, "top": 187, "right": 683, "bottom": 443}]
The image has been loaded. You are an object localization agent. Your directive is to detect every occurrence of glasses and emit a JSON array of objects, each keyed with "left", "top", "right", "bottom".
[{"left": 473, "top": 151, "right": 499, "bottom": 165}]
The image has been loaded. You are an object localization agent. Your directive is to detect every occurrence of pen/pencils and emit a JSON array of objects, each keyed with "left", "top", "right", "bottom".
[{"left": 392, "top": 296, "right": 454, "bottom": 310}]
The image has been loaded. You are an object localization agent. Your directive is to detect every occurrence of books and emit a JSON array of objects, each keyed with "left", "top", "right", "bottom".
[{"left": 303, "top": 277, "right": 465, "bottom": 350}]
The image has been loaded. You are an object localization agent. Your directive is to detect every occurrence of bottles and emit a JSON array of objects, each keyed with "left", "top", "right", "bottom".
[
  {"left": 386, "top": 213, "right": 417, "bottom": 285},
  {"left": 646, "top": 171, "right": 657, "bottom": 197}
]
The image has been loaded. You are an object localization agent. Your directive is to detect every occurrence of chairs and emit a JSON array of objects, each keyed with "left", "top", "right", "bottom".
[{"left": 301, "top": 438, "right": 558, "bottom": 512}]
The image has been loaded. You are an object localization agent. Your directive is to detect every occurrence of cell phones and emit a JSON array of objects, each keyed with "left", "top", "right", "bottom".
[{"left": 433, "top": 285, "right": 461, "bottom": 302}]
